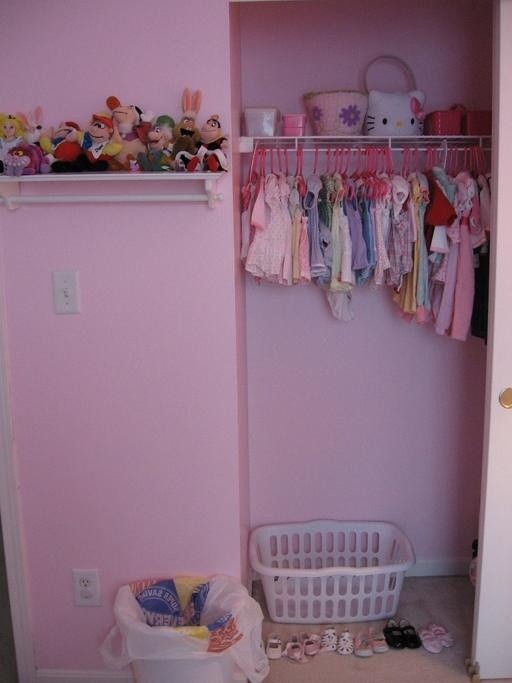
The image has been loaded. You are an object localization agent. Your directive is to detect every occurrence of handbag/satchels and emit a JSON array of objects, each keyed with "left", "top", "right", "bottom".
[{"left": 364, "top": 54, "right": 427, "bottom": 140}]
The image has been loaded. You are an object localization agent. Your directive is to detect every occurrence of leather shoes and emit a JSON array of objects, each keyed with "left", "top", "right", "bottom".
[
  {"left": 383, "top": 616, "right": 406, "bottom": 649},
  {"left": 266, "top": 632, "right": 283, "bottom": 661},
  {"left": 398, "top": 618, "right": 421, "bottom": 649}
]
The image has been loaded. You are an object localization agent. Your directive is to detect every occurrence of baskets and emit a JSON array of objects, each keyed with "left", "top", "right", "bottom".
[
  {"left": 249, "top": 517, "right": 415, "bottom": 626},
  {"left": 301, "top": 88, "right": 368, "bottom": 138}
]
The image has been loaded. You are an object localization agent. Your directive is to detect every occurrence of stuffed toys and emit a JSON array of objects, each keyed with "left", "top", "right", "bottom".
[{"left": 0, "top": 86, "right": 228, "bottom": 176}]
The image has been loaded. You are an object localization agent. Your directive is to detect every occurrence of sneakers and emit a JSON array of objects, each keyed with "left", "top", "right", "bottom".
[
  {"left": 285, "top": 631, "right": 304, "bottom": 660},
  {"left": 299, "top": 631, "right": 320, "bottom": 656},
  {"left": 355, "top": 631, "right": 373, "bottom": 658},
  {"left": 368, "top": 624, "right": 389, "bottom": 654}
]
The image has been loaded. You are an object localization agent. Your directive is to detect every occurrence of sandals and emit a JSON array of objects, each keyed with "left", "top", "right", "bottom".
[
  {"left": 321, "top": 624, "right": 337, "bottom": 652},
  {"left": 338, "top": 628, "right": 354, "bottom": 656},
  {"left": 427, "top": 623, "right": 454, "bottom": 649},
  {"left": 417, "top": 625, "right": 443, "bottom": 654}
]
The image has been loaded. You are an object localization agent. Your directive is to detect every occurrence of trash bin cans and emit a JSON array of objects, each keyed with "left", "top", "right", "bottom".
[{"left": 113, "top": 576, "right": 246, "bottom": 683}]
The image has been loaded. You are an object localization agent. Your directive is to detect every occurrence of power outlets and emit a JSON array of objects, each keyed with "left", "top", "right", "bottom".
[{"left": 73, "top": 569, "right": 99, "bottom": 608}]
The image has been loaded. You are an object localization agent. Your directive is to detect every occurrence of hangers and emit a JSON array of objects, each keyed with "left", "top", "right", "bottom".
[{"left": 246, "top": 144, "right": 491, "bottom": 201}]
